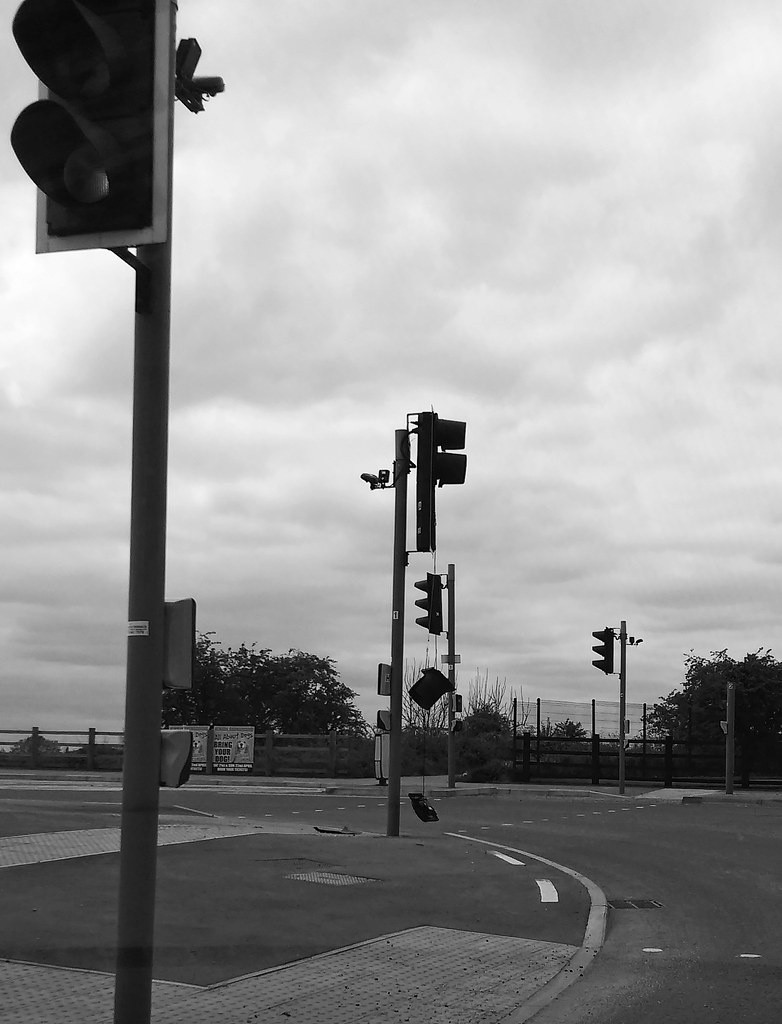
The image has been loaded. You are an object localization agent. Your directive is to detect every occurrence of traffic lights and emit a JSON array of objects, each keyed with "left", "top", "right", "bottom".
[
  {"left": 415, "top": 575, "right": 443, "bottom": 635},
  {"left": 6, "top": 0, "right": 174, "bottom": 252},
  {"left": 420, "top": 412, "right": 469, "bottom": 550},
  {"left": 592, "top": 629, "right": 617, "bottom": 675}
]
